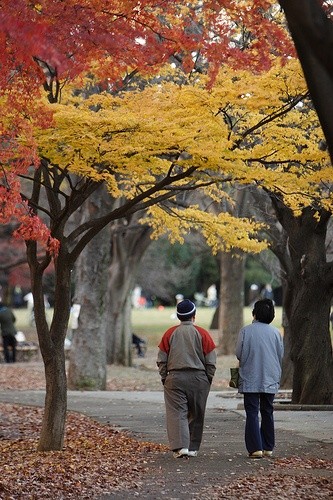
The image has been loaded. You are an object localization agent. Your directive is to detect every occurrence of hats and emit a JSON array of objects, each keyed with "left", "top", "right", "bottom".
[{"left": 177, "top": 299, "right": 196, "bottom": 321}]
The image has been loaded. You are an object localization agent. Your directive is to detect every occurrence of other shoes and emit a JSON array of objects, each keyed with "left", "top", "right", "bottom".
[
  {"left": 263, "top": 450, "right": 272, "bottom": 457},
  {"left": 247, "top": 450, "right": 262, "bottom": 458},
  {"left": 188, "top": 450, "right": 198, "bottom": 457},
  {"left": 173, "top": 448, "right": 188, "bottom": 458}
]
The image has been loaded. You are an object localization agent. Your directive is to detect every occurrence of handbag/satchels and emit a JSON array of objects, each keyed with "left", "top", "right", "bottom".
[{"left": 229, "top": 367, "right": 239, "bottom": 388}]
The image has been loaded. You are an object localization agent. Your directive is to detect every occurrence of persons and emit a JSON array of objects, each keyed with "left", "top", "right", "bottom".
[
  {"left": 0, "top": 301, "right": 17, "bottom": 363},
  {"left": 235, "top": 298, "right": 285, "bottom": 459},
  {"left": 172, "top": 276, "right": 278, "bottom": 311},
  {"left": 22, "top": 281, "right": 157, "bottom": 358},
  {"left": 156, "top": 300, "right": 217, "bottom": 457}
]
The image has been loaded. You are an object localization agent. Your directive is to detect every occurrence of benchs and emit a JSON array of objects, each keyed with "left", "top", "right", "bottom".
[{"left": 0, "top": 343, "right": 147, "bottom": 361}]
